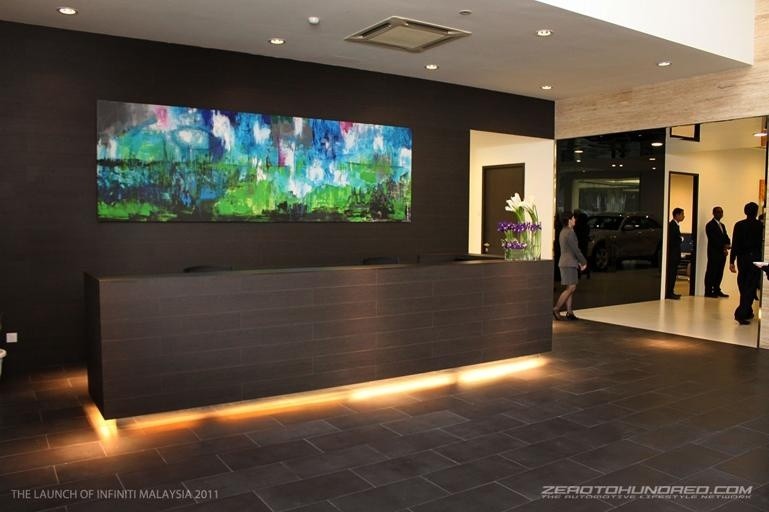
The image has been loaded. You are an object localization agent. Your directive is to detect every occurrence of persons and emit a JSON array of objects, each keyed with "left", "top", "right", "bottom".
[
  {"left": 665, "top": 207, "right": 685, "bottom": 300},
  {"left": 729, "top": 202, "right": 763, "bottom": 324},
  {"left": 704, "top": 207, "right": 729, "bottom": 297},
  {"left": 553, "top": 211, "right": 588, "bottom": 320}
]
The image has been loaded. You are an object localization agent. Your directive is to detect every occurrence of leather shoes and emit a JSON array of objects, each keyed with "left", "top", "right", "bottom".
[
  {"left": 705, "top": 291, "right": 729, "bottom": 298},
  {"left": 665, "top": 293, "right": 681, "bottom": 299},
  {"left": 738, "top": 320, "right": 750, "bottom": 325},
  {"left": 565, "top": 312, "right": 576, "bottom": 321},
  {"left": 553, "top": 309, "right": 561, "bottom": 320}
]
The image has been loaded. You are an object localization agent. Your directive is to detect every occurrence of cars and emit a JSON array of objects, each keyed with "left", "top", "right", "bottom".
[{"left": 579, "top": 211, "right": 663, "bottom": 273}]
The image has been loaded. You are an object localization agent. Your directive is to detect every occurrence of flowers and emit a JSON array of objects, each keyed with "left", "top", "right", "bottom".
[{"left": 497, "top": 193, "right": 542, "bottom": 260}]
